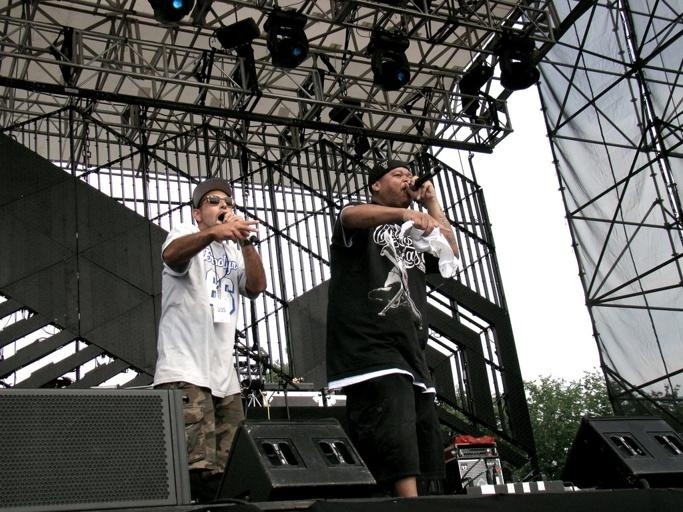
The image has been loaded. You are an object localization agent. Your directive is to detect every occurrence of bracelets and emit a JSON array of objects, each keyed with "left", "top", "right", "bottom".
[{"left": 238, "top": 239, "right": 255, "bottom": 248}]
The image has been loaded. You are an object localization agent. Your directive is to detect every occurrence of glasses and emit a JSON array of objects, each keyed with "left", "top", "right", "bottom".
[{"left": 197, "top": 195, "right": 236, "bottom": 208}]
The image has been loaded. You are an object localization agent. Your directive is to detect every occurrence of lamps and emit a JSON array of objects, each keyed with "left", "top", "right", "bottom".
[{"left": 148, "top": 0, "right": 540, "bottom": 120}]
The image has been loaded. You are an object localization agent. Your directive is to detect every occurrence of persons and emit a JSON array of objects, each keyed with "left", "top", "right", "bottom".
[
  {"left": 329, "top": 159, "right": 461, "bottom": 497},
  {"left": 152, "top": 178, "right": 267, "bottom": 503}
]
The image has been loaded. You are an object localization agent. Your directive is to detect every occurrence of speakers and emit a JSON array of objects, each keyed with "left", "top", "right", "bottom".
[
  {"left": 444, "top": 458, "right": 505, "bottom": 494},
  {"left": 0, "top": 388, "right": 192, "bottom": 512},
  {"left": 561, "top": 415, "right": 683, "bottom": 489},
  {"left": 212, "top": 418, "right": 377, "bottom": 504}
]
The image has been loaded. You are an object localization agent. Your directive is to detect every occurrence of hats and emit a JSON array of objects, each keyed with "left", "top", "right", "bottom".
[
  {"left": 367, "top": 159, "right": 410, "bottom": 194},
  {"left": 192, "top": 177, "right": 231, "bottom": 209}
]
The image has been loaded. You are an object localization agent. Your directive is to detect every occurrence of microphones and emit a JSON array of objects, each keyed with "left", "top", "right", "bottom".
[
  {"left": 409, "top": 166, "right": 442, "bottom": 192},
  {"left": 218, "top": 212, "right": 262, "bottom": 245}
]
